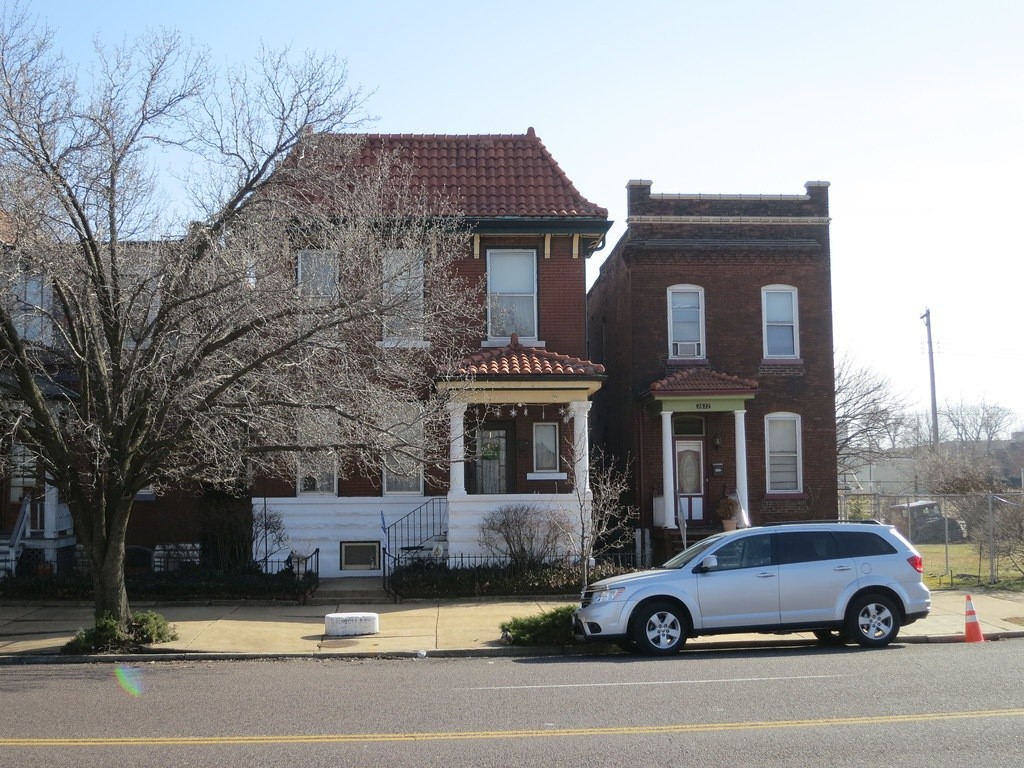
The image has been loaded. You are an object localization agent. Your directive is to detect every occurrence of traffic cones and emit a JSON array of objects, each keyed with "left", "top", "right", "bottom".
[{"left": 963, "top": 593, "right": 991, "bottom": 643}]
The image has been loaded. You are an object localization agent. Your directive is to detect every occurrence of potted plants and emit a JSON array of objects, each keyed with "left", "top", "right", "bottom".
[{"left": 715, "top": 497, "right": 739, "bottom": 531}]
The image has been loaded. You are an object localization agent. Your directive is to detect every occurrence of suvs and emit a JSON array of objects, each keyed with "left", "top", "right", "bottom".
[{"left": 571, "top": 518, "right": 931, "bottom": 658}]
